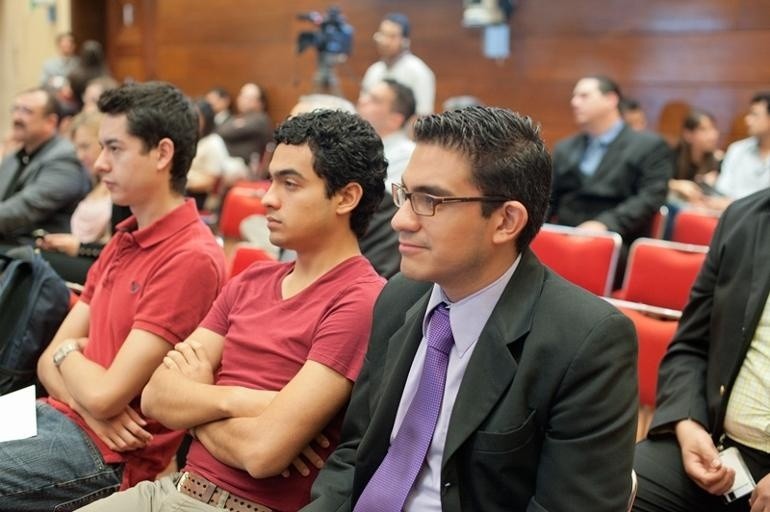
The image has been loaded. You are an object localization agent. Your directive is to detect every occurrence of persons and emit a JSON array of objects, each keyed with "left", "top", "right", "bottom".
[
  {"left": 703, "top": 91, "right": 770, "bottom": 210},
  {"left": 0, "top": 2, "right": 512, "bottom": 285},
  {"left": 73, "top": 109, "right": 389, "bottom": 511},
  {"left": 175, "top": 92, "right": 402, "bottom": 469},
  {"left": 631, "top": 186, "right": 770, "bottom": 511},
  {"left": 618, "top": 99, "right": 646, "bottom": 132},
  {"left": 299, "top": 104, "right": 640, "bottom": 512},
  {"left": 0, "top": 81, "right": 226, "bottom": 512},
  {"left": 544, "top": 76, "right": 675, "bottom": 291},
  {"left": 670, "top": 111, "right": 726, "bottom": 203}
]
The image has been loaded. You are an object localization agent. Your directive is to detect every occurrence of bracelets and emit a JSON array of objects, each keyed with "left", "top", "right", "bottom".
[{"left": 51, "top": 339, "right": 80, "bottom": 368}]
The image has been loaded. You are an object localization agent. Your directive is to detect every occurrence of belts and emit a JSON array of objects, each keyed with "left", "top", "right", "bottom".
[{"left": 176, "top": 470, "right": 271, "bottom": 511}]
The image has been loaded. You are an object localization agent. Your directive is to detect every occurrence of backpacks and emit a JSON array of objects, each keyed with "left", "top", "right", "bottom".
[{"left": 0, "top": 245, "right": 70, "bottom": 395}]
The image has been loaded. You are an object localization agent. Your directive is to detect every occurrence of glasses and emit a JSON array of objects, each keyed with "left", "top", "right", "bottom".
[{"left": 391, "top": 183, "right": 508, "bottom": 215}]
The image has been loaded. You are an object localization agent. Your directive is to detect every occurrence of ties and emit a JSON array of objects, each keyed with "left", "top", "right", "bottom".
[{"left": 352, "top": 305, "right": 454, "bottom": 512}]
